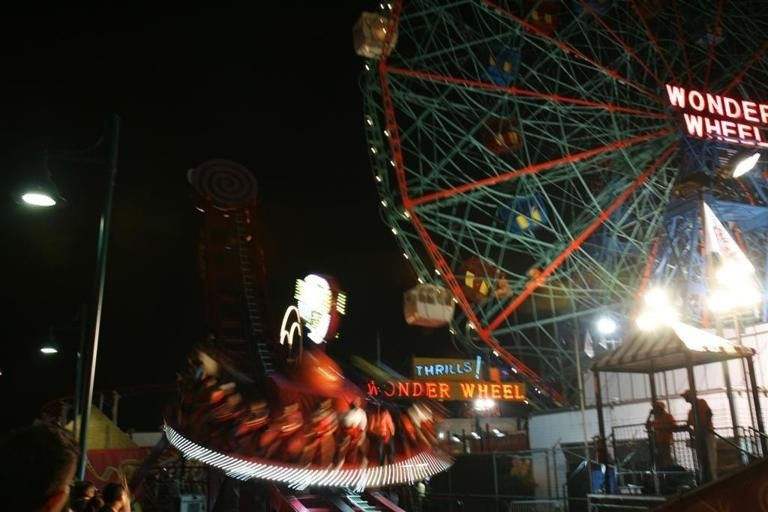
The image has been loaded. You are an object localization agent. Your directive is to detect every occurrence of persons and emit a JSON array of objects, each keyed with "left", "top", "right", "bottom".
[
  {"left": 0, "top": 416, "right": 133, "bottom": 512},
  {"left": 646, "top": 401, "right": 676, "bottom": 468},
  {"left": 681, "top": 391, "right": 719, "bottom": 481}
]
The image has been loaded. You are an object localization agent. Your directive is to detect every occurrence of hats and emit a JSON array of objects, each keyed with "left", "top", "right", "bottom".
[
  {"left": 650, "top": 401, "right": 667, "bottom": 414},
  {"left": 680, "top": 390, "right": 699, "bottom": 400}
]
